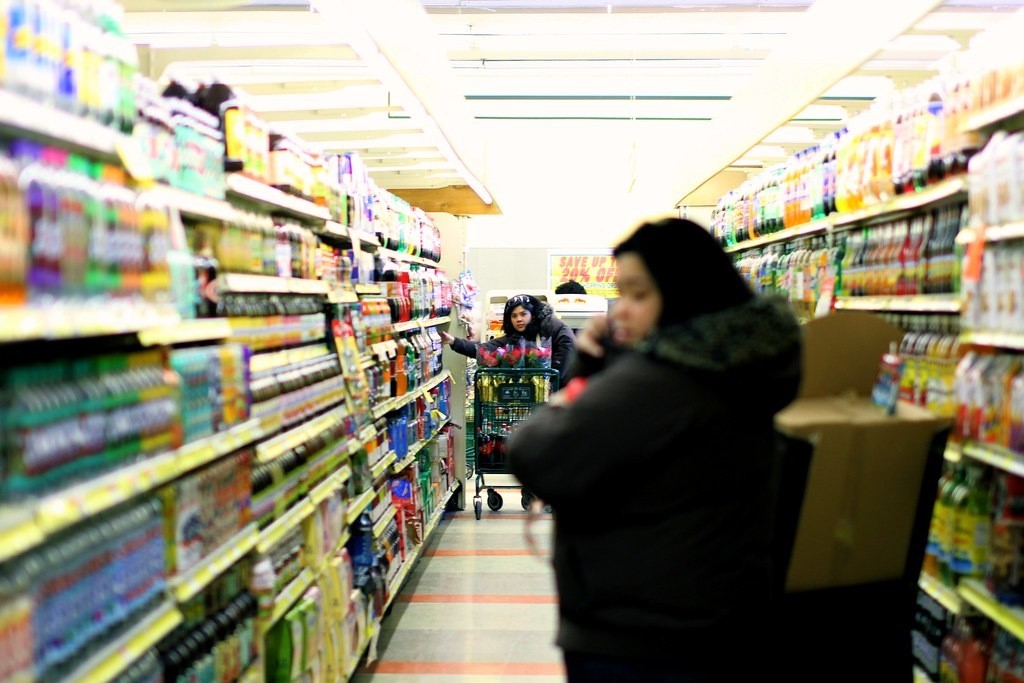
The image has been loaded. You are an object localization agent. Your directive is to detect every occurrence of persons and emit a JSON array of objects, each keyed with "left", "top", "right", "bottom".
[
  {"left": 507, "top": 216, "right": 815, "bottom": 683},
  {"left": 439, "top": 294, "right": 579, "bottom": 393}
]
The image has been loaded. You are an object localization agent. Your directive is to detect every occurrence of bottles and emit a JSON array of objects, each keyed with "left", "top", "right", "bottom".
[
  {"left": 705, "top": 59, "right": 1018, "bottom": 681},
  {"left": 3, "top": 9, "right": 457, "bottom": 681}
]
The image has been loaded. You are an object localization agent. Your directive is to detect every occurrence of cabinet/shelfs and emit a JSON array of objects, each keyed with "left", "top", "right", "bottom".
[
  {"left": 718, "top": 97, "right": 1024, "bottom": 683},
  {"left": 1, "top": 93, "right": 463, "bottom": 683}
]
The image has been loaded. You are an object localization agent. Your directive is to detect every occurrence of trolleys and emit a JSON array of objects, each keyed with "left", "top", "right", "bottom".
[{"left": 474, "top": 367, "right": 559, "bottom": 519}]
